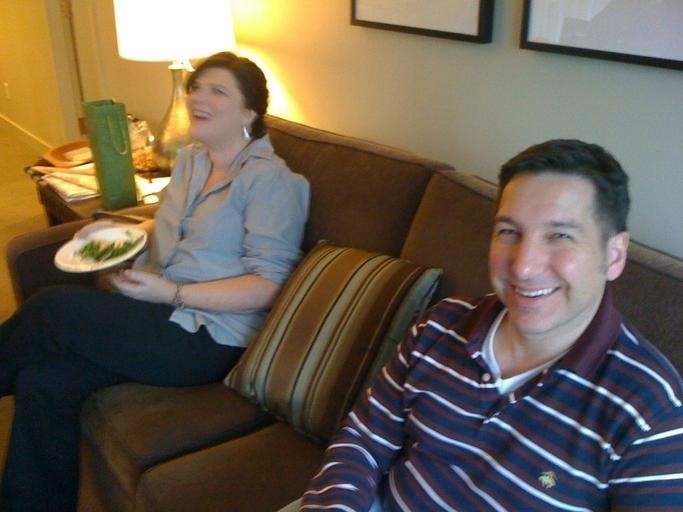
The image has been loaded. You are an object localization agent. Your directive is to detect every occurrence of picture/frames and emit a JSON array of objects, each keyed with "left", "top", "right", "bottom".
[
  {"left": 519, "top": 1, "right": 681, "bottom": 69},
  {"left": 349, "top": 1, "right": 493, "bottom": 45}
]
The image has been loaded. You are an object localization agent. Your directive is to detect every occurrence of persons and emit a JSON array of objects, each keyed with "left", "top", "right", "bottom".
[
  {"left": 0, "top": 50, "right": 311, "bottom": 512},
  {"left": 297, "top": 139, "right": 683, "bottom": 512}
]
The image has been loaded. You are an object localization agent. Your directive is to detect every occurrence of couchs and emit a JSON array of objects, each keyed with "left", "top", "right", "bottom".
[{"left": 7, "top": 121, "right": 681, "bottom": 510}]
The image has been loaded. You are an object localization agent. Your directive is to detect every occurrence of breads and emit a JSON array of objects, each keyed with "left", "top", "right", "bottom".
[{"left": 66, "top": 147, "right": 91, "bottom": 161}]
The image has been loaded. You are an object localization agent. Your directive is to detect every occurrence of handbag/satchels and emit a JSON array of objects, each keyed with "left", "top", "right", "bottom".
[{"left": 81, "top": 98, "right": 138, "bottom": 212}]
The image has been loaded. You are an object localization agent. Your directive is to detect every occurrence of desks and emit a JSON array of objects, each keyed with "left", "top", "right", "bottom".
[{"left": 23, "top": 160, "right": 175, "bottom": 226}]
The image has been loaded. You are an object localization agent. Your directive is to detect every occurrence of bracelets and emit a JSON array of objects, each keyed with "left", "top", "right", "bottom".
[{"left": 173, "top": 282, "right": 184, "bottom": 307}]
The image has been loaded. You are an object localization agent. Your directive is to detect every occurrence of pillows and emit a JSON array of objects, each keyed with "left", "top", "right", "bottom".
[{"left": 218, "top": 197, "right": 447, "bottom": 451}]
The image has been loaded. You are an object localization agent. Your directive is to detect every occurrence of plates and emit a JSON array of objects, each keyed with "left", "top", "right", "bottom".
[{"left": 52, "top": 221, "right": 150, "bottom": 276}]
